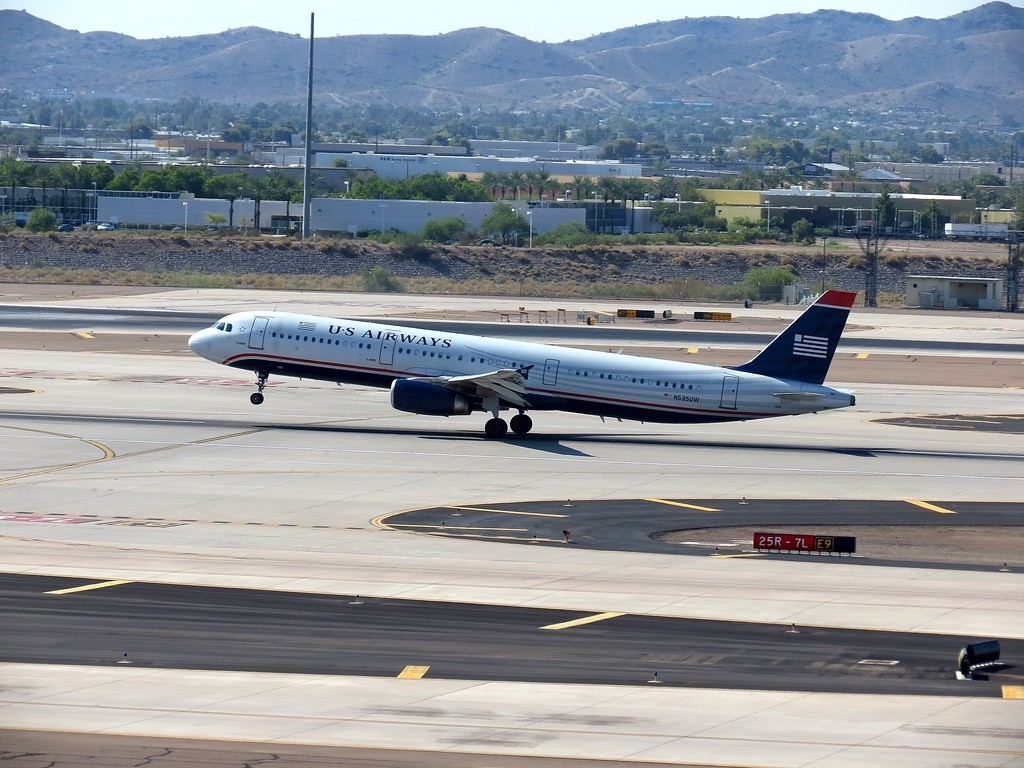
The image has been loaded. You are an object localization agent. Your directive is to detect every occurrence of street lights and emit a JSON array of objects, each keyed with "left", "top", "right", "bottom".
[
  {"left": 526, "top": 211, "right": 533, "bottom": 248},
  {"left": 91, "top": 181, "right": 97, "bottom": 226},
  {"left": 822, "top": 237, "right": 829, "bottom": 293},
  {"left": 479, "top": 239, "right": 501, "bottom": 247},
  {"left": 675, "top": 193, "right": 681, "bottom": 213},
  {"left": 344, "top": 181, "right": 349, "bottom": 194},
  {"left": 595, "top": 195, "right": 602, "bottom": 236},
  {"left": 183, "top": 202, "right": 188, "bottom": 235},
  {"left": 765, "top": 201, "right": 770, "bottom": 232},
  {"left": 512, "top": 208, "right": 518, "bottom": 247}
]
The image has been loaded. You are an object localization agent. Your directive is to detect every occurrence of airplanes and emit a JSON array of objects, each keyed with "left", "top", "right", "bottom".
[{"left": 186, "top": 290, "right": 857, "bottom": 439}]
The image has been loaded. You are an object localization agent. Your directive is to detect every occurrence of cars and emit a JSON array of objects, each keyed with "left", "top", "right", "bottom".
[
  {"left": 97, "top": 223, "right": 115, "bottom": 231},
  {"left": 58, "top": 224, "right": 74, "bottom": 231},
  {"left": 1013, "top": 237, "right": 1024, "bottom": 243},
  {"left": 206, "top": 228, "right": 218, "bottom": 231},
  {"left": 172, "top": 227, "right": 184, "bottom": 233}
]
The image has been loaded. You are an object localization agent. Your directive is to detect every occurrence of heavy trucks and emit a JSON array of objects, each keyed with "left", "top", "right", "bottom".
[{"left": 944, "top": 222, "right": 1016, "bottom": 243}]
